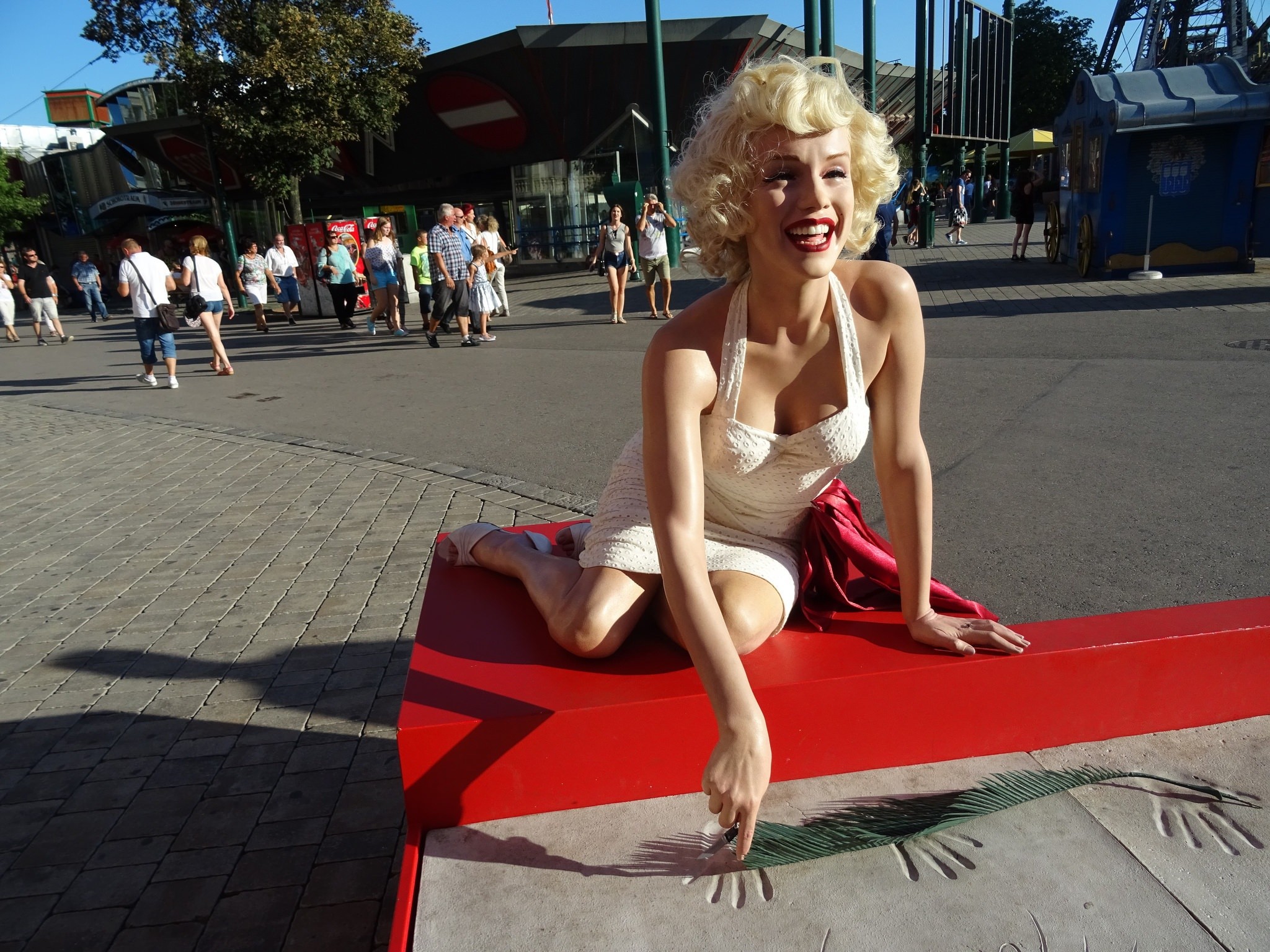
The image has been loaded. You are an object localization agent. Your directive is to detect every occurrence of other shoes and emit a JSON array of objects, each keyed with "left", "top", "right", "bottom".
[
  {"left": 6, "top": 304, "right": 510, "bottom": 346},
  {"left": 902, "top": 235, "right": 918, "bottom": 245},
  {"left": 1011, "top": 255, "right": 1020, "bottom": 262},
  {"left": 1019, "top": 255, "right": 1029, "bottom": 262}
]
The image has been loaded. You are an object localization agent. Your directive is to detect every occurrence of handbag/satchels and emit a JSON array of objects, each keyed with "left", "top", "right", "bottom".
[
  {"left": 486, "top": 250, "right": 496, "bottom": 273},
  {"left": 499, "top": 246, "right": 509, "bottom": 265},
  {"left": 240, "top": 255, "right": 245, "bottom": 282},
  {"left": 597, "top": 260, "right": 607, "bottom": 276},
  {"left": 1031, "top": 185, "right": 1044, "bottom": 204},
  {"left": 953, "top": 208, "right": 966, "bottom": 228},
  {"left": 316, "top": 246, "right": 331, "bottom": 282},
  {"left": 187, "top": 295, "right": 208, "bottom": 320},
  {"left": 157, "top": 303, "right": 179, "bottom": 332}
]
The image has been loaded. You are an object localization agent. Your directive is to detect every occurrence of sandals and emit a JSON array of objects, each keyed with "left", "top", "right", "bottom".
[
  {"left": 436, "top": 522, "right": 553, "bottom": 567},
  {"left": 217, "top": 367, "right": 234, "bottom": 376},
  {"left": 210, "top": 362, "right": 220, "bottom": 371},
  {"left": 555, "top": 523, "right": 593, "bottom": 559},
  {"left": 611, "top": 313, "right": 626, "bottom": 324},
  {"left": 650, "top": 313, "right": 674, "bottom": 319}
]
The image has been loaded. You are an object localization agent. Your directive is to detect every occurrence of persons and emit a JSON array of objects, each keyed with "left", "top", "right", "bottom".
[
  {"left": 0, "top": 194, "right": 677, "bottom": 388},
  {"left": 436, "top": 56, "right": 1030, "bottom": 863},
  {"left": 860, "top": 167, "right": 1043, "bottom": 262}
]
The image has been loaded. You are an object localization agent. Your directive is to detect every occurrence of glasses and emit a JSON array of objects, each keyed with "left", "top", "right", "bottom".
[
  {"left": 967, "top": 174, "right": 971, "bottom": 178},
  {"left": 456, "top": 216, "right": 464, "bottom": 218},
  {"left": 0, "top": 266, "right": 4, "bottom": 268},
  {"left": 27, "top": 254, "right": 37, "bottom": 257},
  {"left": 328, "top": 236, "right": 338, "bottom": 239}
]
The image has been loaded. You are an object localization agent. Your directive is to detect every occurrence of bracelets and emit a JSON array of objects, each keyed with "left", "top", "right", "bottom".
[
  {"left": 330, "top": 266, "right": 333, "bottom": 269},
  {"left": 54, "top": 294, "right": 58, "bottom": 296},
  {"left": 51, "top": 293, "right": 55, "bottom": 296},
  {"left": 445, "top": 276, "right": 450, "bottom": 278},
  {"left": 662, "top": 210, "right": 666, "bottom": 214}
]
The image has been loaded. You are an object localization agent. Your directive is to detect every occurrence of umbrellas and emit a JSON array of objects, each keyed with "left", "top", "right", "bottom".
[{"left": 941, "top": 128, "right": 1056, "bottom": 165}]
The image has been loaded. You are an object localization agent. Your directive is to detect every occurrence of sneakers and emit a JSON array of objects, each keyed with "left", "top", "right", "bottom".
[
  {"left": 945, "top": 233, "right": 953, "bottom": 244},
  {"left": 956, "top": 239, "right": 968, "bottom": 244},
  {"left": 136, "top": 372, "right": 157, "bottom": 386},
  {"left": 168, "top": 380, "right": 178, "bottom": 389}
]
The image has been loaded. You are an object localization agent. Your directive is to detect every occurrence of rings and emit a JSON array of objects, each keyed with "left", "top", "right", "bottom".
[{"left": 965, "top": 623, "right": 971, "bottom": 628}]
